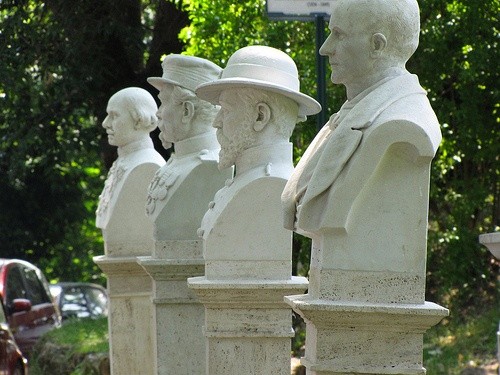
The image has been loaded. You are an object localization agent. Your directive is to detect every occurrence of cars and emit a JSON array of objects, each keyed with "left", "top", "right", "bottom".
[{"left": 0, "top": 258, "right": 109, "bottom": 375}]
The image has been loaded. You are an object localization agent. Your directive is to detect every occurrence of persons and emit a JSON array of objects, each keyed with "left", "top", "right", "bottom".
[
  {"left": 94, "top": 86, "right": 166, "bottom": 229},
  {"left": 280, "top": 0, "right": 442, "bottom": 234},
  {"left": 144, "top": 54, "right": 223, "bottom": 237},
  {"left": 194, "top": 46, "right": 321, "bottom": 262}
]
